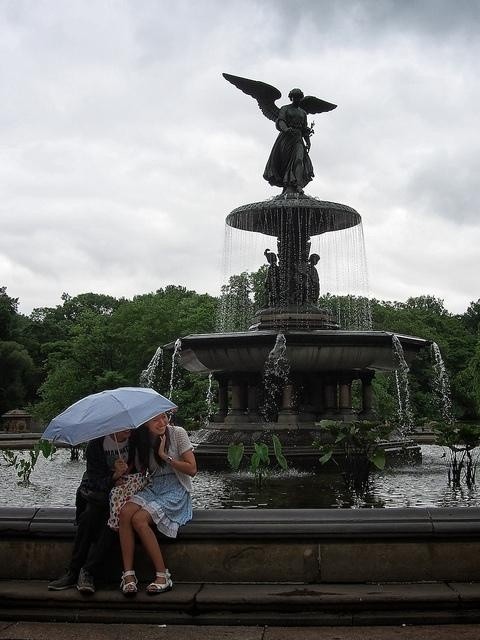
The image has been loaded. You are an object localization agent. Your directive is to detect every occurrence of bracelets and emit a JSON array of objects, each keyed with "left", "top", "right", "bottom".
[{"left": 165, "top": 456, "right": 174, "bottom": 463}]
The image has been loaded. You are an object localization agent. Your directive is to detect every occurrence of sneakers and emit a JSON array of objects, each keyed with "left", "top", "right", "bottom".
[
  {"left": 46, "top": 568, "right": 76, "bottom": 590},
  {"left": 77, "top": 567, "right": 96, "bottom": 593}
]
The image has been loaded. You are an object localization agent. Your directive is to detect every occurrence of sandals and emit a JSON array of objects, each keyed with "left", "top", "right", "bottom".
[
  {"left": 145, "top": 568, "right": 174, "bottom": 595},
  {"left": 119, "top": 570, "right": 140, "bottom": 595}
]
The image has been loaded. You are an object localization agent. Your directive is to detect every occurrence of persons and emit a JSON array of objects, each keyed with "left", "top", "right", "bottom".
[
  {"left": 47, "top": 427, "right": 136, "bottom": 593},
  {"left": 223, "top": 72, "right": 338, "bottom": 195},
  {"left": 263, "top": 247, "right": 279, "bottom": 308},
  {"left": 308, "top": 254, "right": 321, "bottom": 308},
  {"left": 115, "top": 411, "right": 198, "bottom": 596}
]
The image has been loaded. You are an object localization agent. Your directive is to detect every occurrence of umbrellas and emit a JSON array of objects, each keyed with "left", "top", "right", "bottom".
[{"left": 40, "top": 385, "right": 179, "bottom": 459}]
